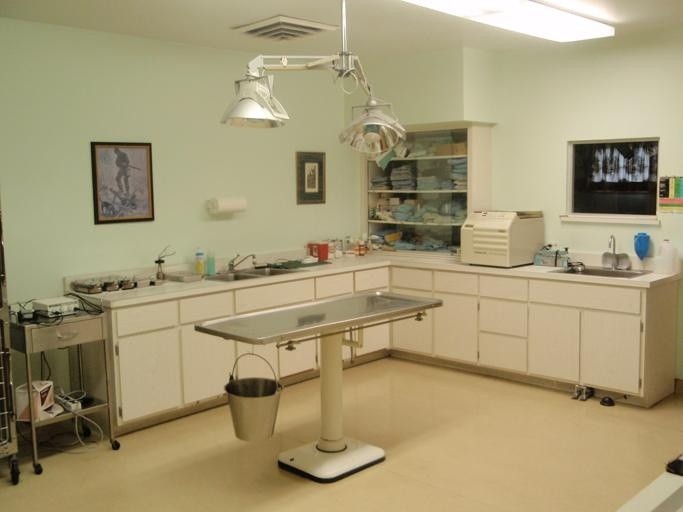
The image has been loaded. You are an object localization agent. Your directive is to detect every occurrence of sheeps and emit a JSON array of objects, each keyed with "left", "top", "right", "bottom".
[
  {"left": 608, "top": 235, "right": 618, "bottom": 272},
  {"left": 228, "top": 254, "right": 256, "bottom": 271}
]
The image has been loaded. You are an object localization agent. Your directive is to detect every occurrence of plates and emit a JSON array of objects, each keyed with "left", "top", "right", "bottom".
[{"left": 360, "top": 119, "right": 497, "bottom": 260}]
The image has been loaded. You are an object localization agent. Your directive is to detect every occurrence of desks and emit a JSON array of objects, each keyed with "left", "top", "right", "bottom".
[{"left": 7, "top": 310, "right": 120, "bottom": 475}]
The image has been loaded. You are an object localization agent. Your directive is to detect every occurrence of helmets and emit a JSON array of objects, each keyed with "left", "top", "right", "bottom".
[{"left": 600, "top": 252, "right": 630, "bottom": 271}]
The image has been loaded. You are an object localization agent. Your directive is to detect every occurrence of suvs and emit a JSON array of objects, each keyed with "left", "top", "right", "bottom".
[{"left": 225, "top": 353, "right": 283, "bottom": 443}]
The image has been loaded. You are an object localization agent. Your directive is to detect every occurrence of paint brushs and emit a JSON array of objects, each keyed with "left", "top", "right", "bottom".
[{"left": 55, "top": 393, "right": 81, "bottom": 412}]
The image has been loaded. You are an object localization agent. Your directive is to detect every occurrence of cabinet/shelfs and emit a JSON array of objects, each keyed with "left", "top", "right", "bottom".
[
  {"left": 62, "top": 254, "right": 394, "bottom": 443},
  {"left": 360, "top": 119, "right": 497, "bottom": 260},
  {"left": 387, "top": 260, "right": 681, "bottom": 409},
  {"left": 358, "top": 241, "right": 365, "bottom": 256}
]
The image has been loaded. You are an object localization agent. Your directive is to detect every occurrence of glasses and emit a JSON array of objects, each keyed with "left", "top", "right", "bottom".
[{"left": 220, "top": 0, "right": 406, "bottom": 155}]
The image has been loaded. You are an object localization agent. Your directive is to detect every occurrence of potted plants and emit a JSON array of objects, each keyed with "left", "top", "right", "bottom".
[
  {"left": 295, "top": 150, "right": 326, "bottom": 206},
  {"left": 90, "top": 140, "right": 154, "bottom": 225}
]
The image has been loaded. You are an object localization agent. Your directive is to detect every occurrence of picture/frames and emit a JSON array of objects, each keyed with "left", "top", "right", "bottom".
[
  {"left": 7, "top": 310, "right": 120, "bottom": 475},
  {"left": 90, "top": 140, "right": 154, "bottom": 225},
  {"left": 295, "top": 150, "right": 326, "bottom": 206}
]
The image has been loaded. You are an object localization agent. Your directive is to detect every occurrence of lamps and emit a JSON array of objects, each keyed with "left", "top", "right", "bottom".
[{"left": 220, "top": 0, "right": 406, "bottom": 155}]
